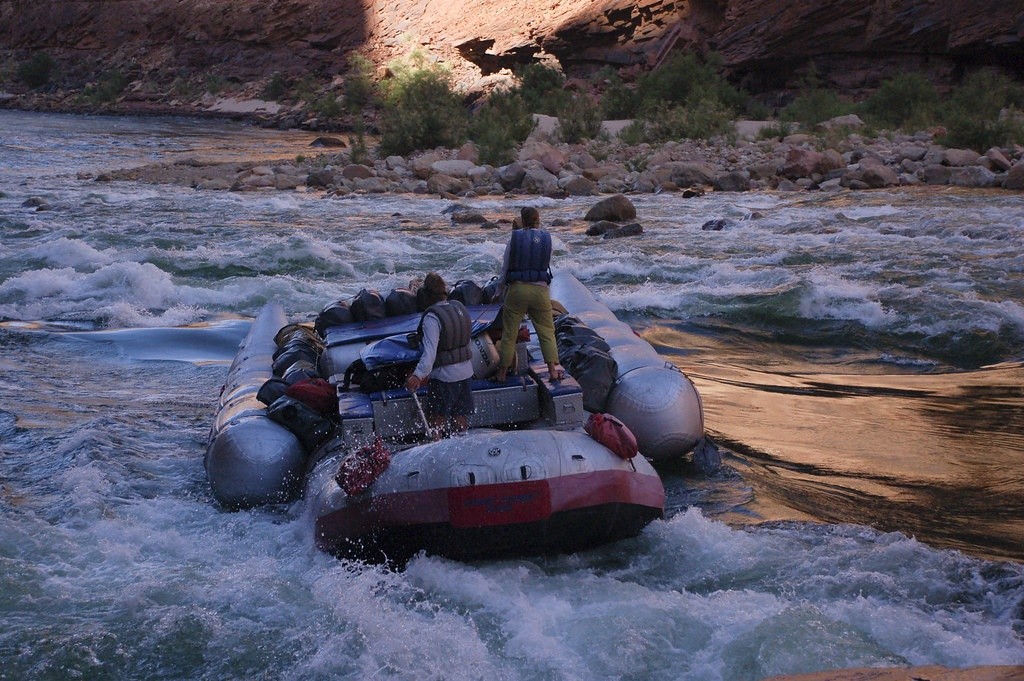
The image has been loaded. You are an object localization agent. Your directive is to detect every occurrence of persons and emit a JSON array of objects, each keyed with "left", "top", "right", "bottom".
[
  {"left": 406, "top": 273, "right": 476, "bottom": 442},
  {"left": 487, "top": 206, "right": 565, "bottom": 384}
]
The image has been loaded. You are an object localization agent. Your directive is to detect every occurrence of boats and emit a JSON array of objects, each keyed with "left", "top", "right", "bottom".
[{"left": 205, "top": 262, "right": 710, "bottom": 564}]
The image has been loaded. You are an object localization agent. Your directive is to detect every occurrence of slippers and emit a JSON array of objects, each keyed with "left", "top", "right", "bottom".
[
  {"left": 548, "top": 370, "right": 565, "bottom": 382},
  {"left": 488, "top": 375, "right": 505, "bottom": 383}
]
